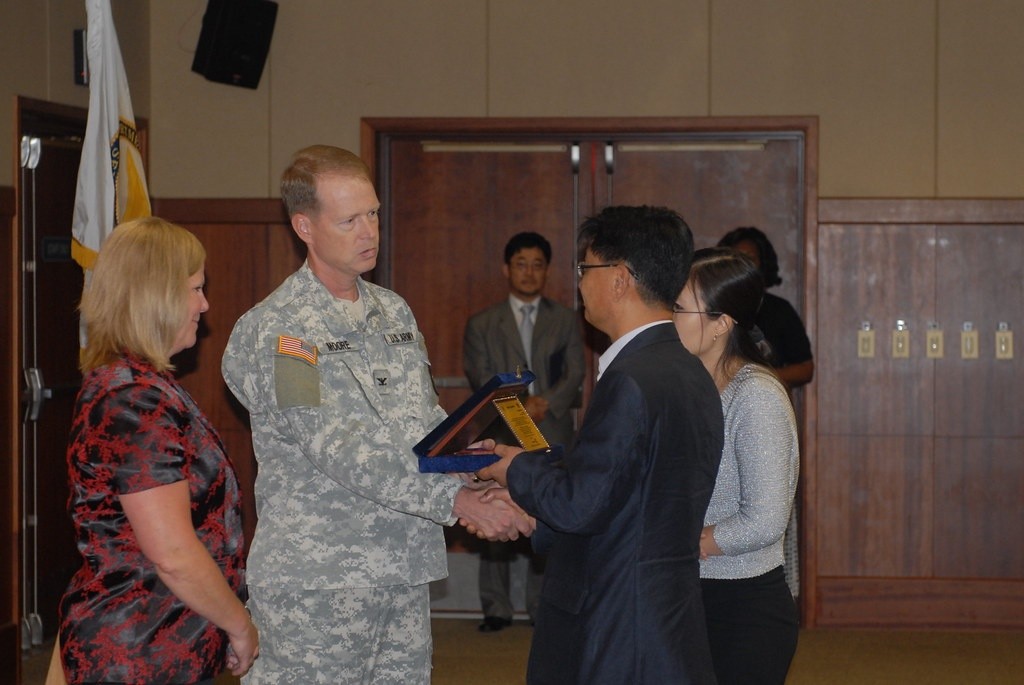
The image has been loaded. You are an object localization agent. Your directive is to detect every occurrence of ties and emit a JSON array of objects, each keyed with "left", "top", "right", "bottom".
[{"left": 519, "top": 305, "right": 536, "bottom": 396}]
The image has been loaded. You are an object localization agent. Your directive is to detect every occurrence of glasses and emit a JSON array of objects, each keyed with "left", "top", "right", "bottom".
[
  {"left": 577, "top": 262, "right": 639, "bottom": 282},
  {"left": 672, "top": 304, "right": 738, "bottom": 325}
]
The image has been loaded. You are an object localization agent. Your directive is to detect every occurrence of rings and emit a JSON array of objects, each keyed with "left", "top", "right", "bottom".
[{"left": 472, "top": 477, "right": 479, "bottom": 483}]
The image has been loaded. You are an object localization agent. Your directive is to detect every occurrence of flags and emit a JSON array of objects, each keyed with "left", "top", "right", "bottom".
[{"left": 70, "top": 0, "right": 154, "bottom": 376}]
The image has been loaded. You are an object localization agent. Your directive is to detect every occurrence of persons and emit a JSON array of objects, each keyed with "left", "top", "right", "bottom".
[
  {"left": 219, "top": 142, "right": 533, "bottom": 685},
  {"left": 58, "top": 215, "right": 261, "bottom": 685},
  {"left": 463, "top": 231, "right": 586, "bottom": 634},
  {"left": 715, "top": 226, "right": 816, "bottom": 601},
  {"left": 459, "top": 204, "right": 727, "bottom": 685},
  {"left": 670, "top": 245, "right": 800, "bottom": 685}
]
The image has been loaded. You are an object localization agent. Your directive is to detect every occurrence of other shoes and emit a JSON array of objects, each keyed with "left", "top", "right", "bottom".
[{"left": 478, "top": 614, "right": 512, "bottom": 631}]
